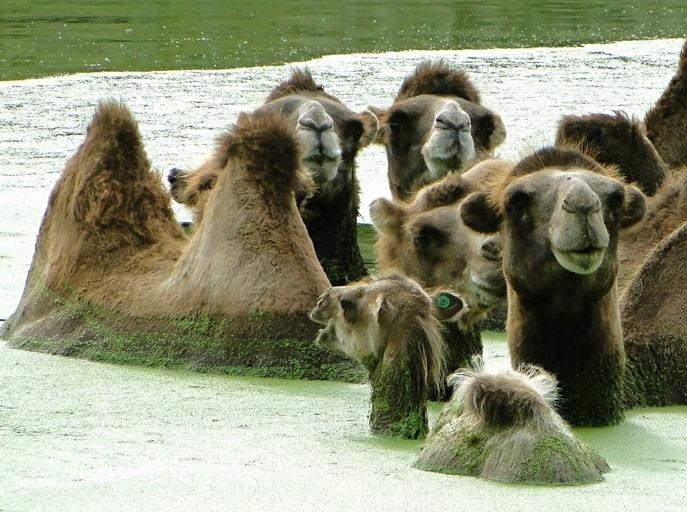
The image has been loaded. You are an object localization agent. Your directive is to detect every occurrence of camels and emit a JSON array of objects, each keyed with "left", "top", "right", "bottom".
[{"left": 369, "top": 36, "right": 686, "bottom": 425}]
